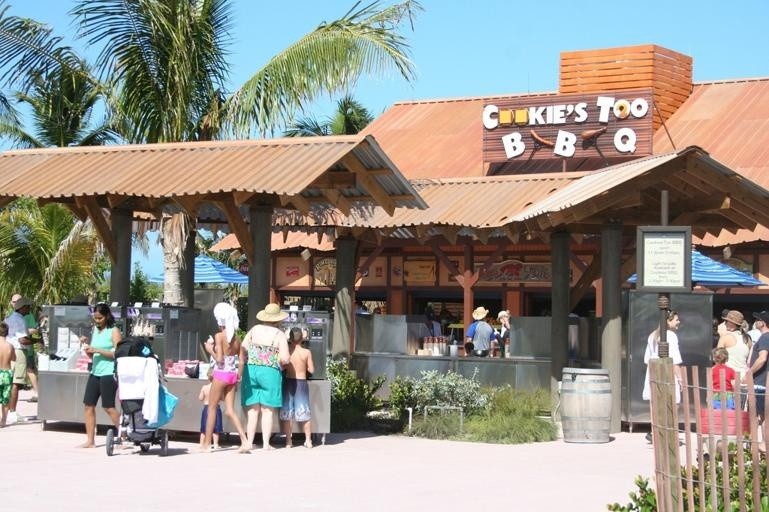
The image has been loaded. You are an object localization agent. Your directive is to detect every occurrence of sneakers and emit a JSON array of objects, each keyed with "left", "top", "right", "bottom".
[{"left": 27, "top": 395, "right": 38, "bottom": 402}]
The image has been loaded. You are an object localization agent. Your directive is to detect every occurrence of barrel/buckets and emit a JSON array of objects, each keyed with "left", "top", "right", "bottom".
[{"left": 559, "top": 366, "right": 614, "bottom": 445}]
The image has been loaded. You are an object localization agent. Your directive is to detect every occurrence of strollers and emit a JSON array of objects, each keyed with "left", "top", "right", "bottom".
[{"left": 105, "top": 334, "right": 179, "bottom": 457}]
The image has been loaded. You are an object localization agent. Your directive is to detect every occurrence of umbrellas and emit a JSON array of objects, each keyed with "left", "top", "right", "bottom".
[
  {"left": 148, "top": 253, "right": 249, "bottom": 289},
  {"left": 626, "top": 250, "right": 768, "bottom": 313}
]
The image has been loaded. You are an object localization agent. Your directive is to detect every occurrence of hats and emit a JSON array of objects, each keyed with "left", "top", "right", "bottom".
[
  {"left": 752, "top": 310, "right": 769, "bottom": 323},
  {"left": 496, "top": 310, "right": 511, "bottom": 323},
  {"left": 471, "top": 306, "right": 489, "bottom": 320},
  {"left": 721, "top": 309, "right": 745, "bottom": 326},
  {"left": 10, "top": 294, "right": 21, "bottom": 304},
  {"left": 14, "top": 297, "right": 35, "bottom": 312},
  {"left": 255, "top": 303, "right": 289, "bottom": 323}
]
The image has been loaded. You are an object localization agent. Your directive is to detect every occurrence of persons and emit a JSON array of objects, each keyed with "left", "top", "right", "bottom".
[
  {"left": 0, "top": 294, "right": 50, "bottom": 428},
  {"left": 639, "top": 307, "right": 767, "bottom": 448},
  {"left": 76, "top": 303, "right": 123, "bottom": 448},
  {"left": 416, "top": 306, "right": 512, "bottom": 358},
  {"left": 194, "top": 303, "right": 315, "bottom": 453}
]
